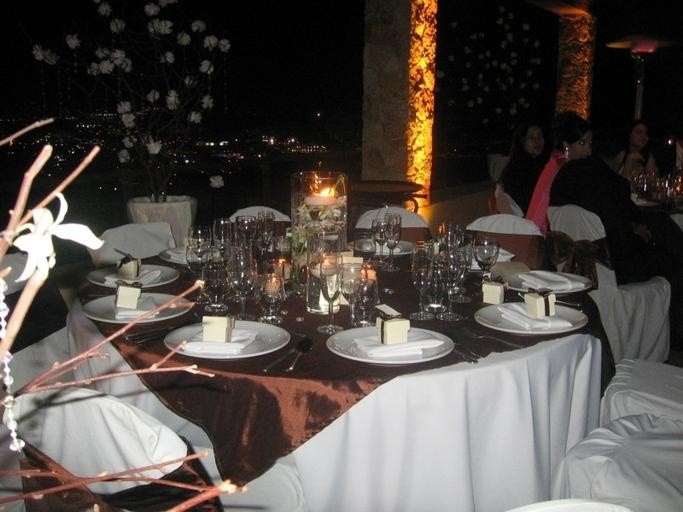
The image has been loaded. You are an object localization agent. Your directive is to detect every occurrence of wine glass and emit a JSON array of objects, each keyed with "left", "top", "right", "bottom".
[{"left": 183, "top": 209, "right": 500, "bottom": 336}]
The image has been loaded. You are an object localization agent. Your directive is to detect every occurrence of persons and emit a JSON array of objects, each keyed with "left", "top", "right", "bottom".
[{"left": 501, "top": 112, "right": 683, "bottom": 352}]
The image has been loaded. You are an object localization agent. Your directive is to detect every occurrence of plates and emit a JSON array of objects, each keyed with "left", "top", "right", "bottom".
[
  {"left": 504, "top": 269, "right": 594, "bottom": 298},
  {"left": 473, "top": 300, "right": 589, "bottom": 337},
  {"left": 81, "top": 241, "right": 195, "bottom": 325},
  {"left": 163, "top": 318, "right": 290, "bottom": 362},
  {"left": 324, "top": 325, "right": 454, "bottom": 368}
]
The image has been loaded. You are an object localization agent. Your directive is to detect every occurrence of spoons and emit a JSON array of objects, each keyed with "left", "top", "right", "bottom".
[{"left": 281, "top": 338, "right": 313, "bottom": 372}]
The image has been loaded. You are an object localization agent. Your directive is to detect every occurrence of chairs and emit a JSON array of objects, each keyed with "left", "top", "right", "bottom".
[
  {"left": 543, "top": 204, "right": 674, "bottom": 365},
  {"left": 547, "top": 406, "right": 680, "bottom": 509},
  {"left": 486, "top": 191, "right": 525, "bottom": 223},
  {"left": 6, "top": 381, "right": 229, "bottom": 512},
  {"left": 602, "top": 351, "right": 681, "bottom": 423}
]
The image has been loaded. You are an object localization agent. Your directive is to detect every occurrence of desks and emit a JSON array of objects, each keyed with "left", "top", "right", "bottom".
[
  {"left": 124, "top": 193, "right": 200, "bottom": 231},
  {"left": 341, "top": 178, "right": 427, "bottom": 218},
  {"left": 56, "top": 207, "right": 608, "bottom": 506}
]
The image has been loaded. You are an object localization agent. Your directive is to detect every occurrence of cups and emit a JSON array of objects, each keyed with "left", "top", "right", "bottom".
[{"left": 627, "top": 168, "right": 682, "bottom": 202}]
